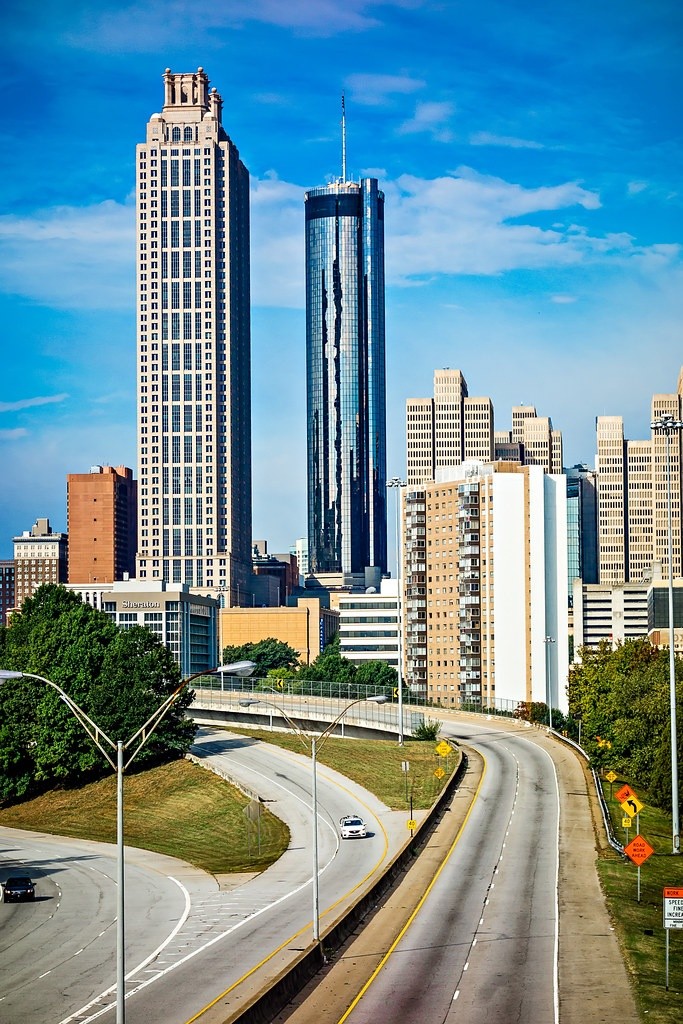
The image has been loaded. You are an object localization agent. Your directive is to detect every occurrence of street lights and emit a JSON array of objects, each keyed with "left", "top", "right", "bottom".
[
  {"left": 238, "top": 696, "right": 388, "bottom": 943},
  {"left": 543, "top": 635, "right": 555, "bottom": 732},
  {"left": 385, "top": 475, "right": 408, "bottom": 746},
  {"left": 651, "top": 410, "right": 683, "bottom": 857},
  {"left": 0, "top": 661, "right": 259, "bottom": 1023}
]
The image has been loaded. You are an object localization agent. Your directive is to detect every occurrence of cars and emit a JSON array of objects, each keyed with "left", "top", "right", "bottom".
[
  {"left": 2, "top": 875, "right": 37, "bottom": 902},
  {"left": 341, "top": 814, "right": 366, "bottom": 839}
]
